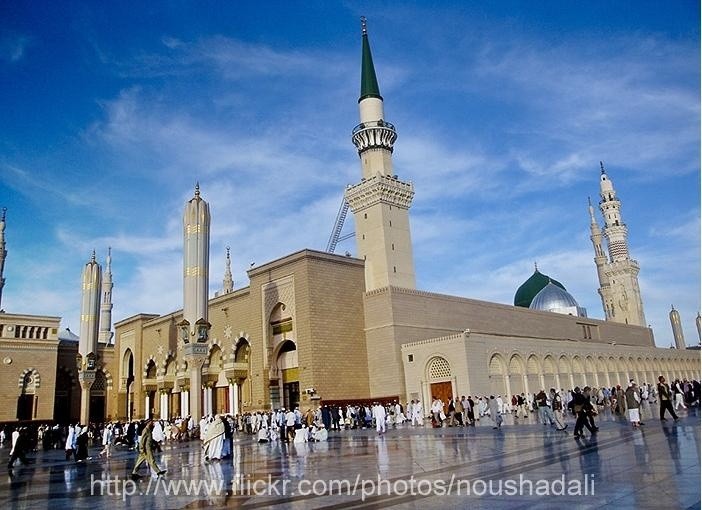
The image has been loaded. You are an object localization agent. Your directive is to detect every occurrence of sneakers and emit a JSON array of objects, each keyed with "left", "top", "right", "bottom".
[
  {"left": 157, "top": 470, "right": 167, "bottom": 476},
  {"left": 86, "top": 457, "right": 92, "bottom": 460},
  {"left": 77, "top": 459, "right": 83, "bottom": 462},
  {"left": 133, "top": 475, "right": 143, "bottom": 478},
  {"left": 555, "top": 425, "right": 568, "bottom": 431},
  {"left": 631, "top": 422, "right": 645, "bottom": 428}
]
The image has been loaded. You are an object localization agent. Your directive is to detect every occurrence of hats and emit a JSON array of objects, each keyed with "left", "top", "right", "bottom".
[{"left": 105, "top": 397, "right": 423, "bottom": 430}]
